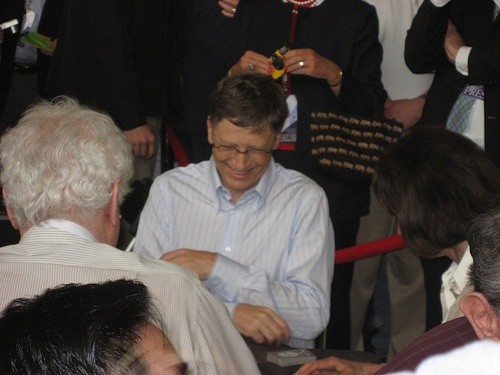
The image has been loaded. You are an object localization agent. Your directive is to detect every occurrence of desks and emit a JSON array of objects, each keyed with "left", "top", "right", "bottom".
[{"left": 240, "top": 333, "right": 388, "bottom": 375}]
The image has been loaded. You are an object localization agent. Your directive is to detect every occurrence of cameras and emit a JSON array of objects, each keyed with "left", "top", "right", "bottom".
[{"left": 268, "top": 49, "right": 288, "bottom": 79}]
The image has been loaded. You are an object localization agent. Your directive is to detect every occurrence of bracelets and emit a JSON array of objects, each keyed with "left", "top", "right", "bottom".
[{"left": 329, "top": 66, "right": 344, "bottom": 88}]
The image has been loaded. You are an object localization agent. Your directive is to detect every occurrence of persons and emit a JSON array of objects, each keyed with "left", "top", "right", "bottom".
[
  {"left": 0, "top": 279, "right": 188, "bottom": 375},
  {"left": 0, "top": 95, "right": 260, "bottom": 374},
  {"left": 136, "top": 69, "right": 339, "bottom": 355},
  {"left": 0, "top": 1, "right": 500, "bottom": 375}
]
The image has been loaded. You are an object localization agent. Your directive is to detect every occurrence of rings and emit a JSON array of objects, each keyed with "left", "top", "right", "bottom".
[{"left": 298, "top": 59, "right": 306, "bottom": 70}]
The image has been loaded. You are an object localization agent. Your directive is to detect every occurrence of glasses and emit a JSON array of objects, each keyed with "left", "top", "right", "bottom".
[{"left": 212, "top": 128, "right": 273, "bottom": 159}]
